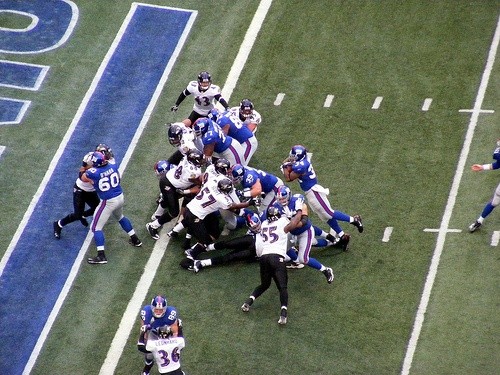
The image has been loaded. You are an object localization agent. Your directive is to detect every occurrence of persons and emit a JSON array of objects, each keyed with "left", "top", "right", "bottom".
[
  {"left": 313, "top": 225, "right": 350, "bottom": 250},
  {"left": 140, "top": 295, "right": 177, "bottom": 375},
  {"left": 230, "top": 99, "right": 261, "bottom": 136},
  {"left": 81, "top": 152, "right": 142, "bottom": 264},
  {"left": 272, "top": 185, "right": 334, "bottom": 284},
  {"left": 54, "top": 144, "right": 112, "bottom": 239},
  {"left": 192, "top": 118, "right": 245, "bottom": 168},
  {"left": 137, "top": 324, "right": 185, "bottom": 375},
  {"left": 282, "top": 145, "right": 363, "bottom": 252},
  {"left": 466, "top": 147, "right": 500, "bottom": 233},
  {"left": 207, "top": 107, "right": 258, "bottom": 166},
  {"left": 240, "top": 203, "right": 307, "bottom": 324},
  {"left": 145, "top": 118, "right": 284, "bottom": 273},
  {"left": 171, "top": 71, "right": 229, "bottom": 129}
]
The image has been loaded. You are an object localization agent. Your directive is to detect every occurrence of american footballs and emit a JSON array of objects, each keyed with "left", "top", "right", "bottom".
[{"left": 286, "top": 164, "right": 293, "bottom": 173}]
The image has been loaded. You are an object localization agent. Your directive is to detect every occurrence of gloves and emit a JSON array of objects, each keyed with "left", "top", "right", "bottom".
[
  {"left": 171, "top": 105, "right": 179, "bottom": 111},
  {"left": 246, "top": 198, "right": 260, "bottom": 206},
  {"left": 82, "top": 151, "right": 94, "bottom": 167},
  {"left": 226, "top": 107, "right": 230, "bottom": 111},
  {"left": 138, "top": 344, "right": 146, "bottom": 354},
  {"left": 176, "top": 188, "right": 184, "bottom": 194}
]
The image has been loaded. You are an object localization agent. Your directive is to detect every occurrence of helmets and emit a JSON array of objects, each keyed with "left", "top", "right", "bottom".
[
  {"left": 218, "top": 179, "right": 232, "bottom": 194},
  {"left": 239, "top": 98, "right": 254, "bottom": 117},
  {"left": 188, "top": 152, "right": 200, "bottom": 166},
  {"left": 231, "top": 165, "right": 244, "bottom": 177},
  {"left": 150, "top": 296, "right": 167, "bottom": 318},
  {"left": 207, "top": 108, "right": 220, "bottom": 122},
  {"left": 198, "top": 71, "right": 212, "bottom": 90},
  {"left": 266, "top": 206, "right": 281, "bottom": 222},
  {"left": 154, "top": 160, "right": 170, "bottom": 175},
  {"left": 89, "top": 143, "right": 112, "bottom": 168},
  {"left": 215, "top": 158, "right": 231, "bottom": 175},
  {"left": 277, "top": 186, "right": 292, "bottom": 205},
  {"left": 157, "top": 325, "right": 171, "bottom": 339},
  {"left": 289, "top": 144, "right": 306, "bottom": 161},
  {"left": 193, "top": 120, "right": 206, "bottom": 135},
  {"left": 168, "top": 125, "right": 182, "bottom": 145}
]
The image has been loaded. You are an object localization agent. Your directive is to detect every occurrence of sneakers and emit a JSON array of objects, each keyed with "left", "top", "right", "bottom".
[
  {"left": 48, "top": 193, "right": 368, "bottom": 324},
  {"left": 469, "top": 221, "right": 481, "bottom": 231},
  {"left": 142, "top": 361, "right": 154, "bottom": 375}
]
